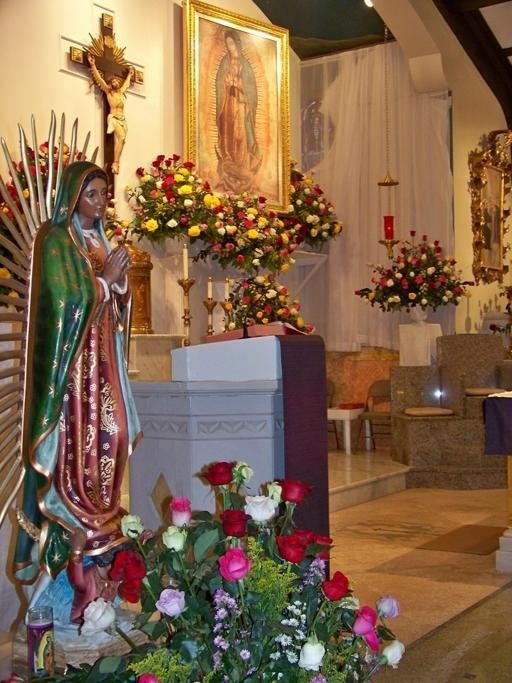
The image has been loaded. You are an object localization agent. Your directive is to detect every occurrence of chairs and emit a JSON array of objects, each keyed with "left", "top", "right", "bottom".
[
  {"left": 358, "top": 380, "right": 392, "bottom": 451},
  {"left": 327, "top": 379, "right": 340, "bottom": 449}
]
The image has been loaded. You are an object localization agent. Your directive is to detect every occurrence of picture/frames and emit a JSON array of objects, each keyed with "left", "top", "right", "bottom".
[{"left": 182, "top": 0, "right": 291, "bottom": 216}]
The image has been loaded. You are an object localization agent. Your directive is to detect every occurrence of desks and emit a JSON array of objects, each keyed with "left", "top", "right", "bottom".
[
  {"left": 327, "top": 407, "right": 365, "bottom": 455},
  {"left": 398, "top": 321, "right": 443, "bottom": 366}
]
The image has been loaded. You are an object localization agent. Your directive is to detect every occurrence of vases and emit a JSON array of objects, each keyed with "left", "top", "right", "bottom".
[
  {"left": 406, "top": 303, "right": 429, "bottom": 326},
  {"left": 165, "top": 234, "right": 189, "bottom": 255},
  {"left": 306, "top": 235, "right": 325, "bottom": 252}
]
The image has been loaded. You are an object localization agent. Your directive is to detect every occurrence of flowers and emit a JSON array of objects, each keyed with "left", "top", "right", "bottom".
[
  {"left": 224, "top": 274, "right": 313, "bottom": 334},
  {"left": 48, "top": 461, "right": 406, "bottom": 683},
  {"left": 354, "top": 229, "right": 474, "bottom": 314},
  {"left": 287, "top": 156, "right": 343, "bottom": 241},
  {"left": 0, "top": 140, "right": 131, "bottom": 312},
  {"left": 190, "top": 191, "right": 302, "bottom": 273},
  {"left": 124, "top": 154, "right": 214, "bottom": 247}
]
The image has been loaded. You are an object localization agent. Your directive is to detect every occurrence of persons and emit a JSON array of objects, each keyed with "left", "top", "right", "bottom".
[
  {"left": 67, "top": 527, "right": 126, "bottom": 623},
  {"left": 87, "top": 53, "right": 136, "bottom": 175},
  {"left": 214, "top": 28, "right": 261, "bottom": 168},
  {"left": 9, "top": 160, "right": 145, "bottom": 586}
]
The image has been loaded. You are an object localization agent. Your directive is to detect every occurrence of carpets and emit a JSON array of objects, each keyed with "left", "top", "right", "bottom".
[
  {"left": 367, "top": 581, "right": 512, "bottom": 682},
  {"left": 415, "top": 525, "right": 509, "bottom": 557}
]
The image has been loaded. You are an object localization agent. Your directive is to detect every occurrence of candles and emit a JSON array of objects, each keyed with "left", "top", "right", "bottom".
[
  {"left": 183, "top": 243, "right": 189, "bottom": 280},
  {"left": 384, "top": 215, "right": 394, "bottom": 240},
  {"left": 224, "top": 277, "right": 230, "bottom": 301},
  {"left": 207, "top": 276, "right": 213, "bottom": 300}
]
